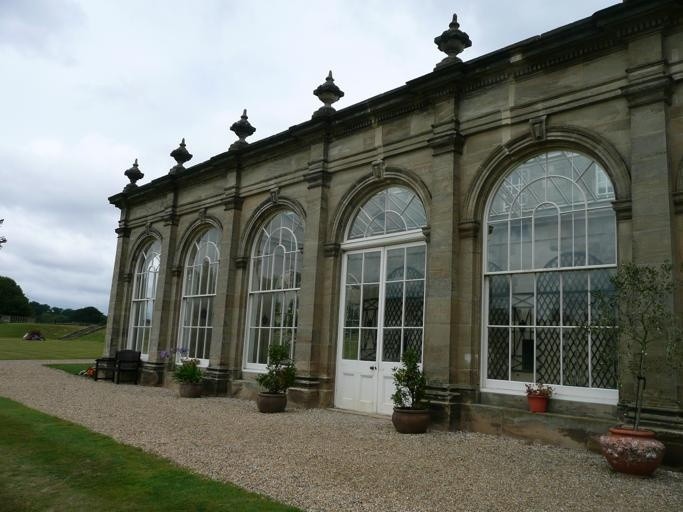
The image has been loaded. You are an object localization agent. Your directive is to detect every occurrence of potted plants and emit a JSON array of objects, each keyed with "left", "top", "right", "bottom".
[
  {"left": 576, "top": 253, "right": 683, "bottom": 476},
  {"left": 254, "top": 339, "right": 299, "bottom": 412},
  {"left": 524, "top": 376, "right": 557, "bottom": 413},
  {"left": 387, "top": 347, "right": 432, "bottom": 434}
]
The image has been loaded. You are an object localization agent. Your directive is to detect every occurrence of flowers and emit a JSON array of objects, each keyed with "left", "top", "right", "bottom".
[{"left": 156, "top": 345, "right": 209, "bottom": 385}]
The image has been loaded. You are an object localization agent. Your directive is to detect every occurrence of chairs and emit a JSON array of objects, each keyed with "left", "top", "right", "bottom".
[{"left": 93, "top": 348, "right": 142, "bottom": 386}]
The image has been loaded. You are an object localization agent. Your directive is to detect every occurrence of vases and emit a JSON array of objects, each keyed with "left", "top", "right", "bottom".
[{"left": 178, "top": 381, "right": 203, "bottom": 398}]
